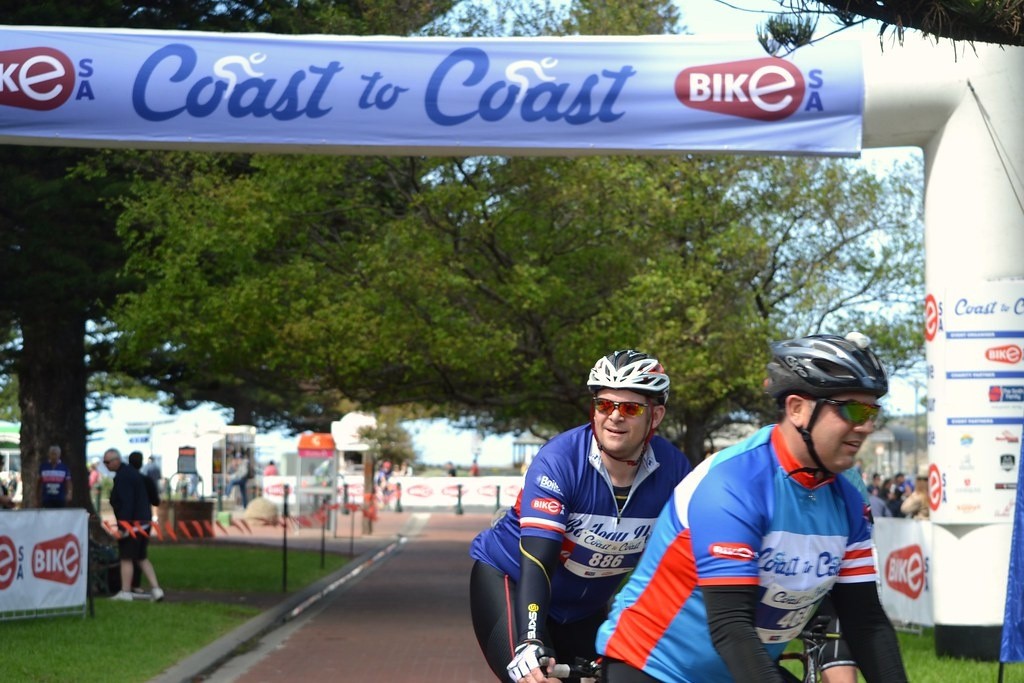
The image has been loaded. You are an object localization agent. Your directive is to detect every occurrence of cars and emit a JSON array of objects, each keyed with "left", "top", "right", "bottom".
[{"left": 0, "top": 448, "right": 26, "bottom": 495}]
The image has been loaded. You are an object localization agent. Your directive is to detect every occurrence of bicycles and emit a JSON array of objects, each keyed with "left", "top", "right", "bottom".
[
  {"left": 376, "top": 485, "right": 397, "bottom": 512},
  {"left": 541, "top": 658, "right": 604, "bottom": 683},
  {"left": 776, "top": 617, "right": 846, "bottom": 683}
]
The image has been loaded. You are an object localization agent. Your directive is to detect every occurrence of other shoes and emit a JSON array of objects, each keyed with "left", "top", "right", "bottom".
[
  {"left": 132, "top": 588, "right": 151, "bottom": 599},
  {"left": 110, "top": 591, "right": 133, "bottom": 602},
  {"left": 150, "top": 587, "right": 164, "bottom": 602}
]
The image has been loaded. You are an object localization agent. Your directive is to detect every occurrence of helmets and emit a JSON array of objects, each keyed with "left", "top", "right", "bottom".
[
  {"left": 765, "top": 331, "right": 888, "bottom": 398},
  {"left": 384, "top": 461, "right": 392, "bottom": 469},
  {"left": 587, "top": 349, "right": 670, "bottom": 406}
]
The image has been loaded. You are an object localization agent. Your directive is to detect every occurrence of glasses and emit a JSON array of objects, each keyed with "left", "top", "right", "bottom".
[
  {"left": 103, "top": 458, "right": 115, "bottom": 465},
  {"left": 593, "top": 393, "right": 649, "bottom": 419},
  {"left": 801, "top": 393, "right": 881, "bottom": 426}
]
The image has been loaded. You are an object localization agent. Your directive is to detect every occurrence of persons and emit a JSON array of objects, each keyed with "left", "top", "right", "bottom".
[
  {"left": 374, "top": 461, "right": 412, "bottom": 509},
  {"left": 103, "top": 449, "right": 198, "bottom": 602},
  {"left": 87, "top": 457, "right": 102, "bottom": 515},
  {"left": 221, "top": 454, "right": 249, "bottom": 507},
  {"left": 468, "top": 350, "right": 691, "bottom": 683},
  {"left": 33, "top": 446, "right": 72, "bottom": 508},
  {"left": 447, "top": 463, "right": 456, "bottom": 477},
  {"left": 0, "top": 454, "right": 23, "bottom": 509},
  {"left": 469, "top": 460, "right": 479, "bottom": 476},
  {"left": 816, "top": 459, "right": 928, "bottom": 683},
  {"left": 264, "top": 461, "right": 278, "bottom": 475},
  {"left": 594, "top": 335, "right": 910, "bottom": 683}
]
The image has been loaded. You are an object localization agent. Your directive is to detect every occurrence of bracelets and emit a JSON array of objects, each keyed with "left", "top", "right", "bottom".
[
  {"left": 67, "top": 490, "right": 71, "bottom": 493},
  {"left": 516, "top": 641, "right": 541, "bottom": 648}
]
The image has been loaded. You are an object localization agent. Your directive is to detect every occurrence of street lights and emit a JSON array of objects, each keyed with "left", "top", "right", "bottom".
[{"left": 914, "top": 379, "right": 920, "bottom": 476}]
